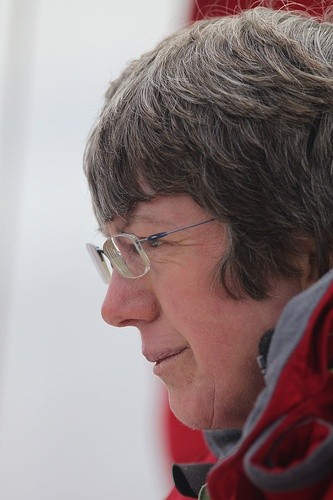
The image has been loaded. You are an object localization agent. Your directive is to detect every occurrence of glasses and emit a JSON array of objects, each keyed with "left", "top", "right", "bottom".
[{"left": 86, "top": 218, "right": 216, "bottom": 285}]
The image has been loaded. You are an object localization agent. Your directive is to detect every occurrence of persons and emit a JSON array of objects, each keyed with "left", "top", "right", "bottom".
[{"left": 81, "top": 6, "right": 333, "bottom": 500}]
